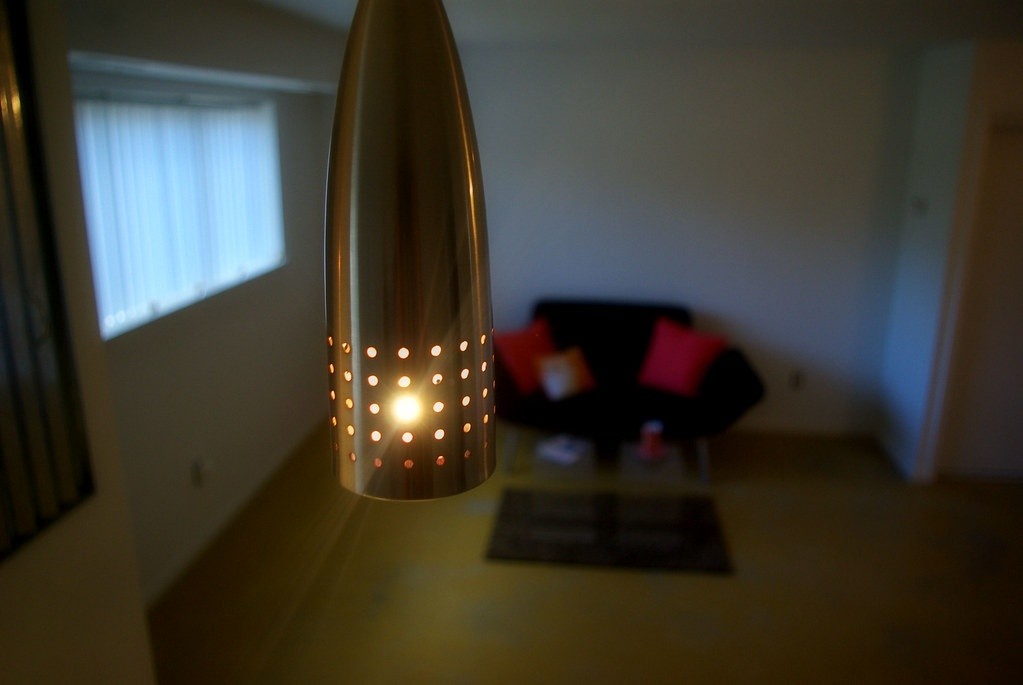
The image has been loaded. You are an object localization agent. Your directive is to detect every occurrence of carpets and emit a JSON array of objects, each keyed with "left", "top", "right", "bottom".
[{"left": 482, "top": 486, "right": 733, "bottom": 576}]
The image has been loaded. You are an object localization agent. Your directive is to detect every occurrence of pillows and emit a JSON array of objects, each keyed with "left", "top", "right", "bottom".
[
  {"left": 532, "top": 347, "right": 595, "bottom": 401},
  {"left": 490, "top": 318, "right": 556, "bottom": 396},
  {"left": 640, "top": 320, "right": 728, "bottom": 396}
]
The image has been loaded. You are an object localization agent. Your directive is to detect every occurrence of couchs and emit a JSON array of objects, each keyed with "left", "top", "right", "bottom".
[{"left": 476, "top": 286, "right": 764, "bottom": 465}]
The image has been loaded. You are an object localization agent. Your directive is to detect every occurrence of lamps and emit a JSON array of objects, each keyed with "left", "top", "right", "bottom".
[{"left": 324, "top": 0, "right": 501, "bottom": 501}]
